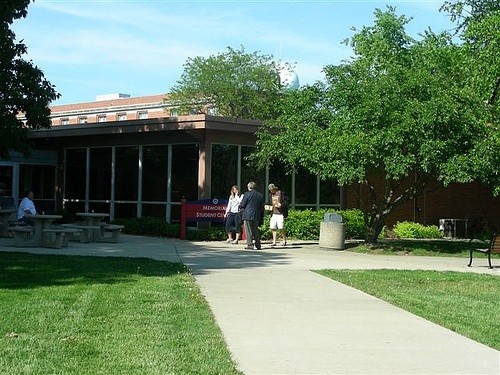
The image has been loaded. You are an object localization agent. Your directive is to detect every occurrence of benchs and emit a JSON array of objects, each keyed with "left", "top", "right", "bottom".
[
  {"left": 7, "top": 219, "right": 124, "bottom": 249},
  {"left": 468, "top": 232, "right": 500, "bottom": 269}
]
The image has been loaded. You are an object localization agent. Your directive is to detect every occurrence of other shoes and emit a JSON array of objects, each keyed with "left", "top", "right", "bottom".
[
  {"left": 244, "top": 245, "right": 254, "bottom": 250},
  {"left": 230, "top": 239, "right": 238, "bottom": 244},
  {"left": 279, "top": 242, "right": 287, "bottom": 246},
  {"left": 226, "top": 239, "right": 233, "bottom": 243},
  {"left": 257, "top": 246, "right": 261, "bottom": 250},
  {"left": 270, "top": 242, "right": 275, "bottom": 246}
]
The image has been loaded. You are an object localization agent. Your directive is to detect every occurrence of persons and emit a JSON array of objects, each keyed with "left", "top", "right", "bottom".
[
  {"left": 240, "top": 181, "right": 265, "bottom": 250},
  {"left": 268, "top": 183, "right": 289, "bottom": 246},
  {"left": 223, "top": 185, "right": 244, "bottom": 244},
  {"left": 17, "top": 190, "right": 39, "bottom": 236}
]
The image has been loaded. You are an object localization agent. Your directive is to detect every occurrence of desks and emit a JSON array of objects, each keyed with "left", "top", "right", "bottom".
[
  {"left": 23, "top": 215, "right": 62, "bottom": 246},
  {"left": 75, "top": 213, "right": 110, "bottom": 244}
]
